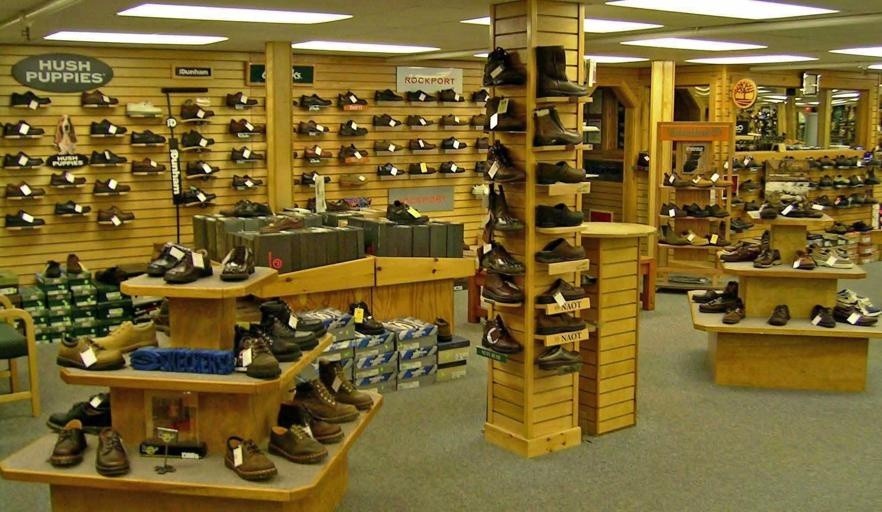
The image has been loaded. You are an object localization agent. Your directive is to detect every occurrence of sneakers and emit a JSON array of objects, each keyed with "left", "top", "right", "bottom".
[{"left": 812, "top": 288, "right": 882, "bottom": 327}]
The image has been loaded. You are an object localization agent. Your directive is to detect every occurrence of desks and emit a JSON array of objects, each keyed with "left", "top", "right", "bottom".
[{"left": 575, "top": 221, "right": 659, "bottom": 438}]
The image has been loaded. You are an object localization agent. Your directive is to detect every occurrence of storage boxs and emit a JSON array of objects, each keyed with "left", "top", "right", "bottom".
[
  {"left": 195, "top": 206, "right": 466, "bottom": 275},
  {"left": 303, "top": 308, "right": 470, "bottom": 394},
  {"left": 843, "top": 228, "right": 882, "bottom": 266},
  {"left": 1, "top": 270, "right": 160, "bottom": 343}
]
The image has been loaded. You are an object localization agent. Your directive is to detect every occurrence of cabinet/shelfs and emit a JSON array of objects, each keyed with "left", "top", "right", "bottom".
[
  {"left": 688, "top": 211, "right": 882, "bottom": 394},
  {"left": 742, "top": 150, "right": 881, "bottom": 210},
  {"left": 0, "top": 264, "right": 382, "bottom": 512},
  {"left": 654, "top": 119, "right": 732, "bottom": 302}
]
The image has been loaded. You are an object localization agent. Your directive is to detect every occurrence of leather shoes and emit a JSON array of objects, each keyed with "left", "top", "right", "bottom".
[
  {"left": 95, "top": 267, "right": 128, "bottom": 285},
  {"left": 46, "top": 260, "right": 60, "bottom": 278},
  {"left": 147, "top": 243, "right": 255, "bottom": 282},
  {"left": 307, "top": 197, "right": 360, "bottom": 211},
  {"left": 56, "top": 297, "right": 170, "bottom": 371},
  {"left": 2, "top": 92, "right": 166, "bottom": 230},
  {"left": 226, "top": 301, "right": 373, "bottom": 479},
  {"left": 347, "top": 303, "right": 385, "bottom": 335},
  {"left": 67, "top": 255, "right": 82, "bottom": 273},
  {"left": 692, "top": 280, "right": 744, "bottom": 322},
  {"left": 260, "top": 216, "right": 303, "bottom": 234},
  {"left": 300, "top": 88, "right": 486, "bottom": 186},
  {"left": 48, "top": 392, "right": 129, "bottom": 476},
  {"left": 178, "top": 92, "right": 272, "bottom": 217},
  {"left": 482, "top": 45, "right": 588, "bottom": 370},
  {"left": 387, "top": 201, "right": 429, "bottom": 225},
  {"left": 770, "top": 304, "right": 789, "bottom": 324},
  {"left": 433, "top": 317, "right": 452, "bottom": 342},
  {"left": 657, "top": 154, "right": 881, "bottom": 269}
]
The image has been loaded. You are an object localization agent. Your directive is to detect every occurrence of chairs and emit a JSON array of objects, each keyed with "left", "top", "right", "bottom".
[
  {"left": 590, "top": 208, "right": 658, "bottom": 312},
  {"left": 0, "top": 294, "right": 42, "bottom": 420}
]
